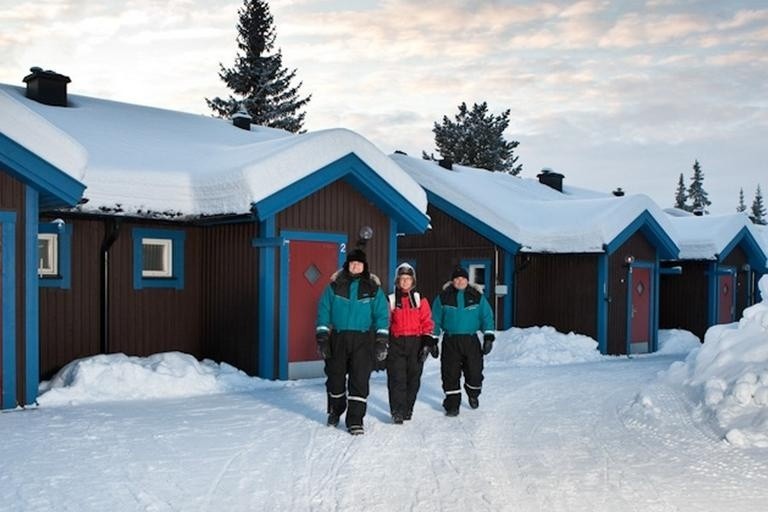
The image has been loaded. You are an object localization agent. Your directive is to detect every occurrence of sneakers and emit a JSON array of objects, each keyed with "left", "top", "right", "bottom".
[
  {"left": 327, "top": 403, "right": 347, "bottom": 427},
  {"left": 394, "top": 407, "right": 412, "bottom": 424},
  {"left": 348, "top": 424, "right": 364, "bottom": 435},
  {"left": 469, "top": 396, "right": 479, "bottom": 409},
  {"left": 445, "top": 408, "right": 458, "bottom": 417}
]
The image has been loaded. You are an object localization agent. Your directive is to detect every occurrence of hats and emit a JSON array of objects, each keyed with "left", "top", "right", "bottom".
[
  {"left": 343, "top": 249, "right": 369, "bottom": 275},
  {"left": 394, "top": 262, "right": 417, "bottom": 289},
  {"left": 451, "top": 265, "right": 469, "bottom": 281}
]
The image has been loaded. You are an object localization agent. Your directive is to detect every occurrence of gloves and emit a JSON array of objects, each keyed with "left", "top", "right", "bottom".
[
  {"left": 418, "top": 334, "right": 434, "bottom": 363},
  {"left": 374, "top": 332, "right": 390, "bottom": 362},
  {"left": 431, "top": 338, "right": 439, "bottom": 359},
  {"left": 315, "top": 332, "right": 332, "bottom": 360},
  {"left": 482, "top": 334, "right": 495, "bottom": 355}
]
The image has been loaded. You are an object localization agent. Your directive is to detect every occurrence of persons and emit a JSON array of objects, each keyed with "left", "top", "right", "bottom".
[
  {"left": 429, "top": 266, "right": 496, "bottom": 418},
  {"left": 384, "top": 261, "right": 434, "bottom": 425},
  {"left": 314, "top": 249, "right": 392, "bottom": 436}
]
